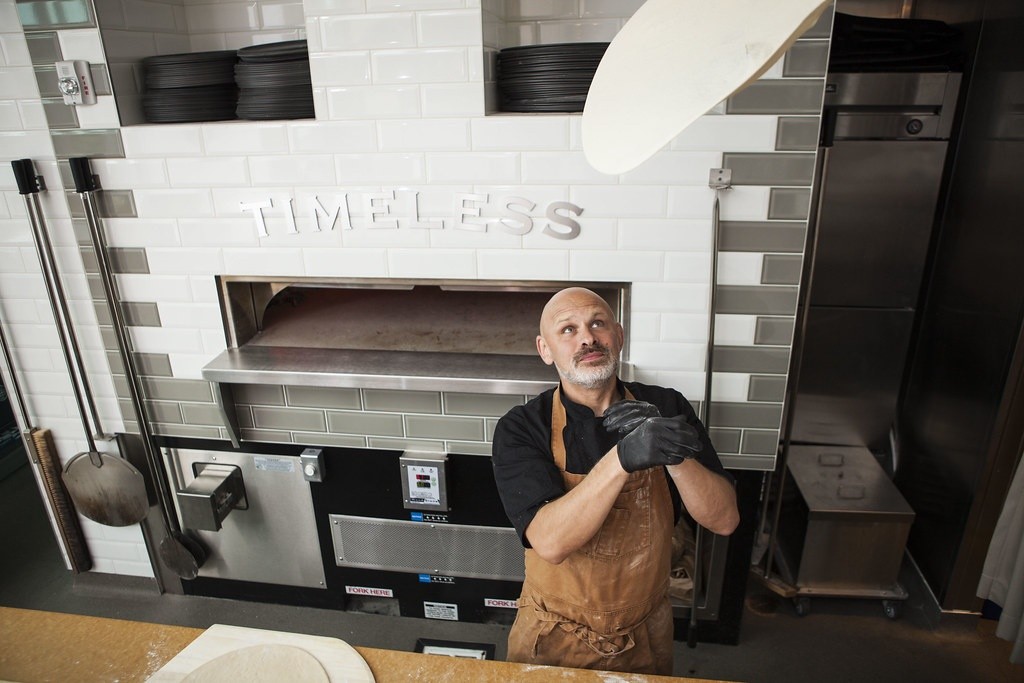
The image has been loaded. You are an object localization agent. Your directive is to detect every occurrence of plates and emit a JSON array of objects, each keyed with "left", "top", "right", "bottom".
[
  {"left": 494, "top": 41, "right": 615, "bottom": 113},
  {"left": 138, "top": 39, "right": 315, "bottom": 124}
]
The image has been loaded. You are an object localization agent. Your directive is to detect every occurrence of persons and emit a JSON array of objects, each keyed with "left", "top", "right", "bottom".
[{"left": 492, "top": 287, "right": 740, "bottom": 676}]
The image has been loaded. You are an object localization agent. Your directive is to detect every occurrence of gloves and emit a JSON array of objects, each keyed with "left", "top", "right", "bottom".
[
  {"left": 617, "top": 413, "right": 702, "bottom": 472},
  {"left": 602, "top": 399, "right": 663, "bottom": 435}
]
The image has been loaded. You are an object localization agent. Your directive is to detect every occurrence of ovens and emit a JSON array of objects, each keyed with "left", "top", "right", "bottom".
[{"left": 750, "top": 63, "right": 967, "bottom": 621}]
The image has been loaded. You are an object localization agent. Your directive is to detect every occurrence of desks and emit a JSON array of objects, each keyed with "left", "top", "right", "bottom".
[{"left": 0, "top": 605, "right": 732, "bottom": 682}]
